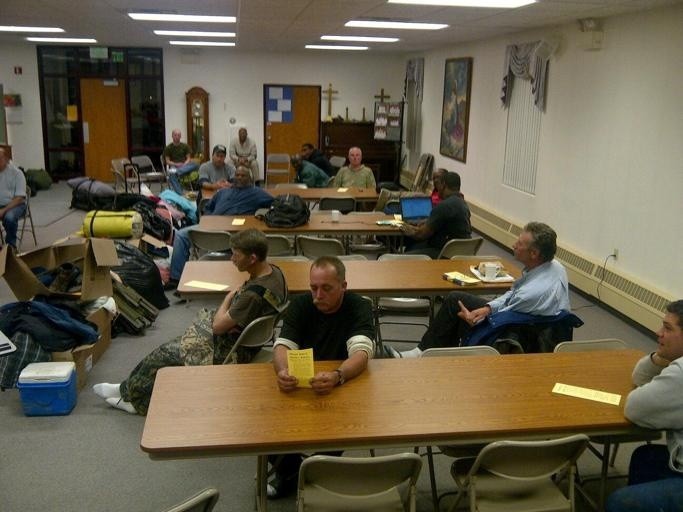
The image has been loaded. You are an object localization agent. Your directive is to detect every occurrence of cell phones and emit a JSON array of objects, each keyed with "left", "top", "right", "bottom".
[{"left": 376, "top": 221, "right": 391, "bottom": 225}]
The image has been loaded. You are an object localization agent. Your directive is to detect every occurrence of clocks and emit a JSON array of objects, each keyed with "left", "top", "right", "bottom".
[{"left": 184, "top": 86, "right": 212, "bottom": 164}]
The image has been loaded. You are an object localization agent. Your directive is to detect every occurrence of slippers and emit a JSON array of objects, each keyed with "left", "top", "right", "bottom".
[{"left": 383, "top": 344, "right": 404, "bottom": 359}]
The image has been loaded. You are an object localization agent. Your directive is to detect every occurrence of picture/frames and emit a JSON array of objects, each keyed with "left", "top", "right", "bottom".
[{"left": 437, "top": 56, "right": 475, "bottom": 165}]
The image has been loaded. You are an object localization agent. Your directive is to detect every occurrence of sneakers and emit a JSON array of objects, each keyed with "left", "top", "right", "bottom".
[{"left": 268, "top": 475, "right": 296, "bottom": 501}]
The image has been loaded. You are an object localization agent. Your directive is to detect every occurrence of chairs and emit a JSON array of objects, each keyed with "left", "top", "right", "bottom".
[
  {"left": 1, "top": 185, "right": 37, "bottom": 254},
  {"left": 141, "top": 254, "right": 681, "bottom": 512},
  {"left": 112, "top": 153, "right": 483, "bottom": 261}
]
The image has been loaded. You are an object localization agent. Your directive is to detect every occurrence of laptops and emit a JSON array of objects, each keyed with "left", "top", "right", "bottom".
[{"left": 401, "top": 196, "right": 434, "bottom": 226}]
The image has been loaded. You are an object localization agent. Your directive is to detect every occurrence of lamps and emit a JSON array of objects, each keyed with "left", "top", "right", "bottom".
[{"left": 572, "top": 14, "right": 605, "bottom": 53}]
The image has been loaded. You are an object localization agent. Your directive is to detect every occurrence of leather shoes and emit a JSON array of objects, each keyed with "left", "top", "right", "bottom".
[{"left": 164, "top": 280, "right": 178, "bottom": 291}]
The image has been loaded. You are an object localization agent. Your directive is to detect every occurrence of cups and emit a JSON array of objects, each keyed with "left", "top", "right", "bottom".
[
  {"left": 331, "top": 209, "right": 342, "bottom": 223},
  {"left": 483, "top": 263, "right": 501, "bottom": 281}
]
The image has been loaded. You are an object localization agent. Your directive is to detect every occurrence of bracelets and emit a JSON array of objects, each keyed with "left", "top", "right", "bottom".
[{"left": 333, "top": 369, "right": 344, "bottom": 385}]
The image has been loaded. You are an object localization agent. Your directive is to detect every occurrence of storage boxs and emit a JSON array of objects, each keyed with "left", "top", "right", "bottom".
[
  {"left": 17, "top": 361, "right": 78, "bottom": 418},
  {"left": 1, "top": 235, "right": 166, "bottom": 398}
]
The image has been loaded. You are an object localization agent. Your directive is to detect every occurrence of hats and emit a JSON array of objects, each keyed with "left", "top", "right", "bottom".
[{"left": 213, "top": 145, "right": 226, "bottom": 157}]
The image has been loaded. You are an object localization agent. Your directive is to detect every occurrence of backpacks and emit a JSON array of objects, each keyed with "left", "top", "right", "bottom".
[{"left": 265, "top": 194, "right": 310, "bottom": 228}]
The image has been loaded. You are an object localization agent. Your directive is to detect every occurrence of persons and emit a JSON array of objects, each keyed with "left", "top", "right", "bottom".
[
  {"left": 384, "top": 222, "right": 571, "bottom": 358},
  {"left": 606, "top": 300, "right": 683, "bottom": 512},
  {"left": 0, "top": 144, "right": 28, "bottom": 254},
  {"left": 93, "top": 227, "right": 291, "bottom": 416},
  {"left": 267, "top": 256, "right": 375, "bottom": 500}
]
